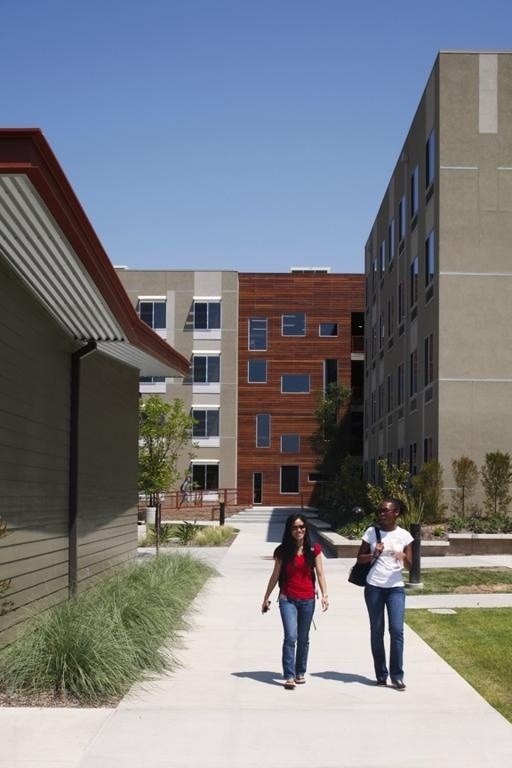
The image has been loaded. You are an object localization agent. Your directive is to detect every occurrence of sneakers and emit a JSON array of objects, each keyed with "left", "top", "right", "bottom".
[
  {"left": 390, "top": 677, "right": 407, "bottom": 690},
  {"left": 376, "top": 679, "right": 387, "bottom": 687},
  {"left": 295, "top": 674, "right": 307, "bottom": 685},
  {"left": 284, "top": 679, "right": 296, "bottom": 691}
]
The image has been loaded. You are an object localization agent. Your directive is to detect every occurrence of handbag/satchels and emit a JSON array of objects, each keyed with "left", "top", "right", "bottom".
[{"left": 347, "top": 556, "right": 370, "bottom": 588}]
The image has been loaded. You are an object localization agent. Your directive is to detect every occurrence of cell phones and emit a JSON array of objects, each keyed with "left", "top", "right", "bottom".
[{"left": 263, "top": 600, "right": 271, "bottom": 612}]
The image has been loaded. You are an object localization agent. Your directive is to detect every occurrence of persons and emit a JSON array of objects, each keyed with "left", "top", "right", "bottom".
[
  {"left": 261, "top": 512, "right": 329, "bottom": 690},
  {"left": 357, "top": 497, "right": 415, "bottom": 690}
]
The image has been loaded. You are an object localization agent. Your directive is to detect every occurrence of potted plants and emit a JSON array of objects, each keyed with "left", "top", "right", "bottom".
[{"left": 139, "top": 393, "right": 200, "bottom": 522}]
